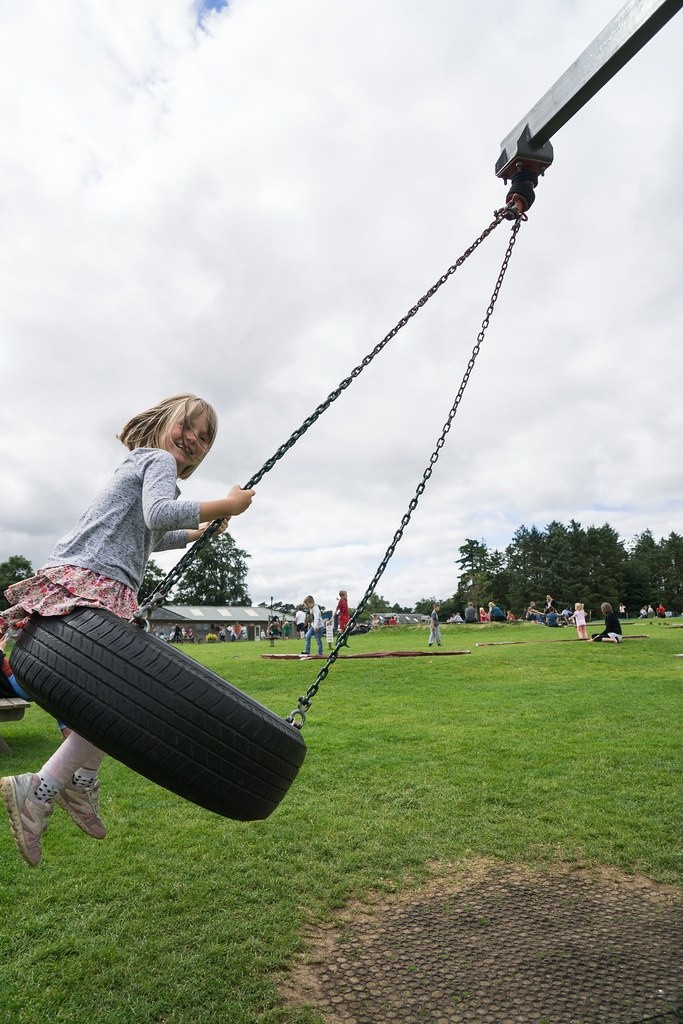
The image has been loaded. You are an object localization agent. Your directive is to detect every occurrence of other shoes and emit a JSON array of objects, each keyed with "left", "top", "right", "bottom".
[
  {"left": 438, "top": 643, "right": 442, "bottom": 646},
  {"left": 429, "top": 643, "right": 433, "bottom": 646},
  {"left": 615, "top": 637, "right": 620, "bottom": 644},
  {"left": 301, "top": 651, "right": 309, "bottom": 654}
]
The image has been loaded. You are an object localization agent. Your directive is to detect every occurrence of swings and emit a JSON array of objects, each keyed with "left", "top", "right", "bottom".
[{"left": 9, "top": 207, "right": 525, "bottom": 823}]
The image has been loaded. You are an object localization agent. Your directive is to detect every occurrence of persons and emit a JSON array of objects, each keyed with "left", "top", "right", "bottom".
[
  {"left": 0, "top": 618, "right": 74, "bottom": 741},
  {"left": 0, "top": 392, "right": 254, "bottom": 868},
  {"left": 587, "top": 602, "right": 623, "bottom": 644},
  {"left": 568, "top": 603, "right": 588, "bottom": 640},
  {"left": 152, "top": 591, "right": 668, "bottom": 655},
  {"left": 426, "top": 604, "right": 442, "bottom": 647}
]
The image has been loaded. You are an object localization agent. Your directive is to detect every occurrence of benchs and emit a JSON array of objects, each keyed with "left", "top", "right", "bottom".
[{"left": 0, "top": 698, "right": 31, "bottom": 757}]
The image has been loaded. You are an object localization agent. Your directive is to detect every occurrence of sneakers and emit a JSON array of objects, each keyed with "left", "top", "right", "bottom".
[
  {"left": 0, "top": 772, "right": 56, "bottom": 866},
  {"left": 53, "top": 773, "right": 107, "bottom": 839}
]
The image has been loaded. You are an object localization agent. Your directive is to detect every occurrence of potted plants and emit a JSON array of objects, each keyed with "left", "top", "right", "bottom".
[{"left": 205, "top": 633, "right": 218, "bottom": 643}]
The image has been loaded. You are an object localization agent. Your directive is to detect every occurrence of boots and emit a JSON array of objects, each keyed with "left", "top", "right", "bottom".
[
  {"left": 335, "top": 633, "right": 342, "bottom": 646},
  {"left": 343, "top": 634, "right": 349, "bottom": 647}
]
